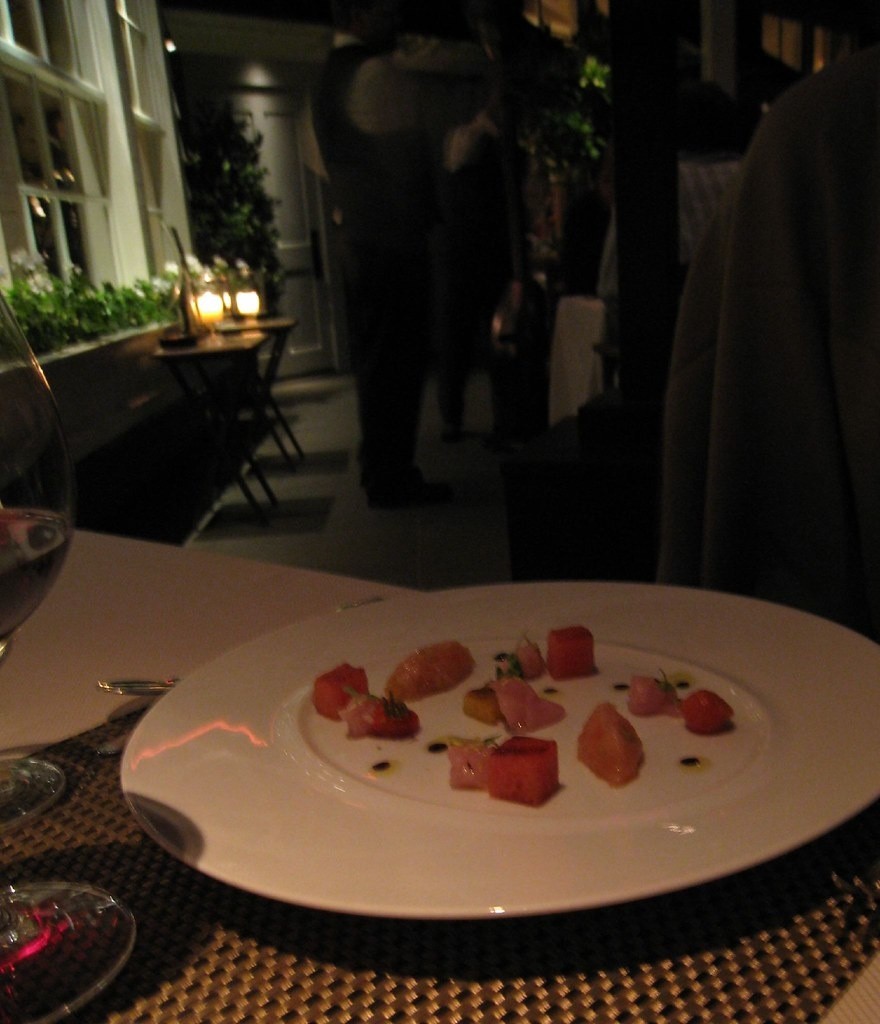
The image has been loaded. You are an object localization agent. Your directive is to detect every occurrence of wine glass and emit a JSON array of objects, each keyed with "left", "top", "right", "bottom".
[
  {"left": 2, "top": 879, "right": 134, "bottom": 1024},
  {"left": 0, "top": 286, "right": 77, "bottom": 828}
]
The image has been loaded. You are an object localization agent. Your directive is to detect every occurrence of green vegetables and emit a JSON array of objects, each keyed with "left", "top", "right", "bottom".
[{"left": 345, "top": 630, "right": 681, "bottom": 743}]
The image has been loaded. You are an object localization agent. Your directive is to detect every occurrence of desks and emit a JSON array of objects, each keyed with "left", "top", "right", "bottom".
[
  {"left": 207, "top": 317, "right": 304, "bottom": 472},
  {"left": 151, "top": 331, "right": 279, "bottom": 520}
]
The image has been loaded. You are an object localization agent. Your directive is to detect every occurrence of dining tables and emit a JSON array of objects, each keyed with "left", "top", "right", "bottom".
[{"left": 1, "top": 528, "right": 879, "bottom": 1023}]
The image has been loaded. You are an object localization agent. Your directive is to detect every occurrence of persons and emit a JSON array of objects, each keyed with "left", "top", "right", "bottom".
[{"left": 312, "top": 0, "right": 880, "bottom": 643}]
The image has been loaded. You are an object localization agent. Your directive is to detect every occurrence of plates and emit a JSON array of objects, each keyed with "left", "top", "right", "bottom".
[{"left": 122, "top": 582, "right": 879, "bottom": 920}]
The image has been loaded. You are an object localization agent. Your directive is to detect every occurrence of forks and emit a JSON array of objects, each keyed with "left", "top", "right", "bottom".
[{"left": 96, "top": 679, "right": 174, "bottom": 696}]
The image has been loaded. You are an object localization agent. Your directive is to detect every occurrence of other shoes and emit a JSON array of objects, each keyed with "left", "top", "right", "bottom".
[
  {"left": 367, "top": 480, "right": 456, "bottom": 509},
  {"left": 439, "top": 426, "right": 471, "bottom": 442},
  {"left": 360, "top": 465, "right": 380, "bottom": 486}
]
